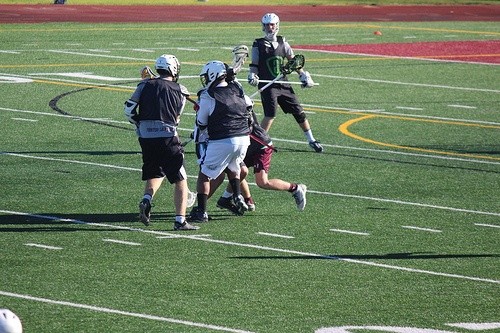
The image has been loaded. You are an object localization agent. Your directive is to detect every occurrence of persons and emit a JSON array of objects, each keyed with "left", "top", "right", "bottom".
[
  {"left": 124, "top": 54, "right": 199, "bottom": 230},
  {"left": 184, "top": 61, "right": 250, "bottom": 222},
  {"left": 248, "top": 14, "right": 323, "bottom": 153},
  {"left": 195, "top": 54, "right": 307, "bottom": 212}
]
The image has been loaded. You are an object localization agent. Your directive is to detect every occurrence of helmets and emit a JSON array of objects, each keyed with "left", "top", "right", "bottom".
[
  {"left": 154, "top": 54, "right": 182, "bottom": 83},
  {"left": 260, "top": 12, "right": 280, "bottom": 40},
  {"left": 199, "top": 60, "right": 228, "bottom": 88},
  {"left": 0, "top": 308, "right": 23, "bottom": 333}
]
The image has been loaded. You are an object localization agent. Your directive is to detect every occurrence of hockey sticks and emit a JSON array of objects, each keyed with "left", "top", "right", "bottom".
[
  {"left": 141, "top": 66, "right": 278, "bottom": 153},
  {"left": 230, "top": 45, "right": 249, "bottom": 74},
  {"left": 182, "top": 55, "right": 306, "bottom": 149},
  {"left": 234, "top": 77, "right": 321, "bottom": 86}
]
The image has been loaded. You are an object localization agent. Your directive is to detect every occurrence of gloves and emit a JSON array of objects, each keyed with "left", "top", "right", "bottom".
[
  {"left": 247, "top": 72, "right": 259, "bottom": 87},
  {"left": 298, "top": 71, "right": 315, "bottom": 88}
]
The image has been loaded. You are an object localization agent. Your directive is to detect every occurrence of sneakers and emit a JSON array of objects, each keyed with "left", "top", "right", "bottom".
[
  {"left": 216, "top": 192, "right": 256, "bottom": 216},
  {"left": 173, "top": 219, "right": 201, "bottom": 231},
  {"left": 308, "top": 140, "right": 323, "bottom": 153},
  {"left": 138, "top": 198, "right": 155, "bottom": 226},
  {"left": 187, "top": 209, "right": 209, "bottom": 223},
  {"left": 290, "top": 184, "right": 307, "bottom": 212}
]
